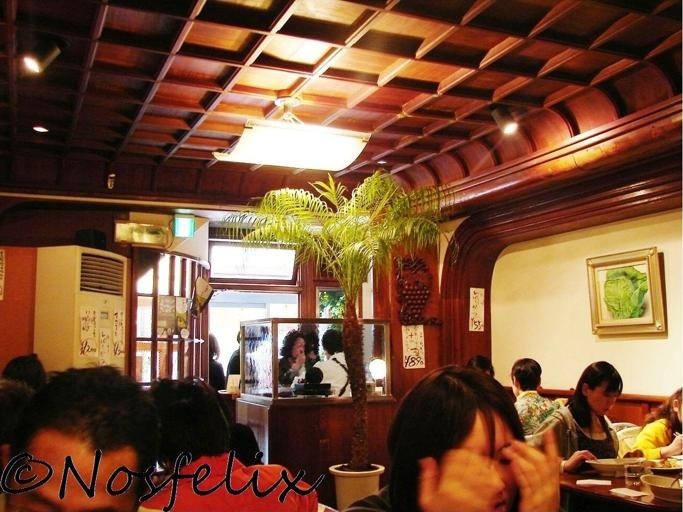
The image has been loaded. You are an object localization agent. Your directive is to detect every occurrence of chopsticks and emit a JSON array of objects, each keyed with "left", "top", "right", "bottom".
[{"left": 672, "top": 432, "right": 680, "bottom": 437}]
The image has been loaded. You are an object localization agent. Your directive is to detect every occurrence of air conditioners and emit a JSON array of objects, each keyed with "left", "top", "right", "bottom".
[{"left": 32, "top": 244, "right": 127, "bottom": 378}]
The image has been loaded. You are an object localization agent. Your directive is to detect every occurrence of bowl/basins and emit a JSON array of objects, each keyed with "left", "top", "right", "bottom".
[
  {"left": 638, "top": 472, "right": 682, "bottom": 504},
  {"left": 585, "top": 457, "right": 638, "bottom": 477}
]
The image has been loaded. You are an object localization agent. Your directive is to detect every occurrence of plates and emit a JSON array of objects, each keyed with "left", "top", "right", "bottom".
[
  {"left": 668, "top": 455, "right": 682, "bottom": 461},
  {"left": 637, "top": 460, "right": 683, "bottom": 472}
]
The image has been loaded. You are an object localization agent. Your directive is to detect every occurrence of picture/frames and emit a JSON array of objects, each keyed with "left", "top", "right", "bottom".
[{"left": 583, "top": 244, "right": 670, "bottom": 336}]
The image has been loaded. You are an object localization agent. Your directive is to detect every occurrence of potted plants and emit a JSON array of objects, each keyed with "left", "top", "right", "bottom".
[{"left": 220, "top": 162, "right": 448, "bottom": 512}]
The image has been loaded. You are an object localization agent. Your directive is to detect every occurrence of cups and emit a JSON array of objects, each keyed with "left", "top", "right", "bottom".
[
  {"left": 645, "top": 458, "right": 665, "bottom": 478},
  {"left": 622, "top": 459, "right": 649, "bottom": 486}
]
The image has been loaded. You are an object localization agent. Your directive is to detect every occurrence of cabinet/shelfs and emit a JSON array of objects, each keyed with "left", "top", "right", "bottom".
[{"left": 235, "top": 315, "right": 399, "bottom": 512}]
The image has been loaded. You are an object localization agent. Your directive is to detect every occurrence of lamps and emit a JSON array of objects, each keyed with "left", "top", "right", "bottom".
[
  {"left": 212, "top": 94, "right": 374, "bottom": 172},
  {"left": 23, "top": 40, "right": 62, "bottom": 74},
  {"left": 490, "top": 106, "right": 519, "bottom": 135}
]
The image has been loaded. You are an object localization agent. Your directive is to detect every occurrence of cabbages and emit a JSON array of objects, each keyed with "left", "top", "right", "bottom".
[{"left": 603, "top": 266, "right": 647, "bottom": 320}]
